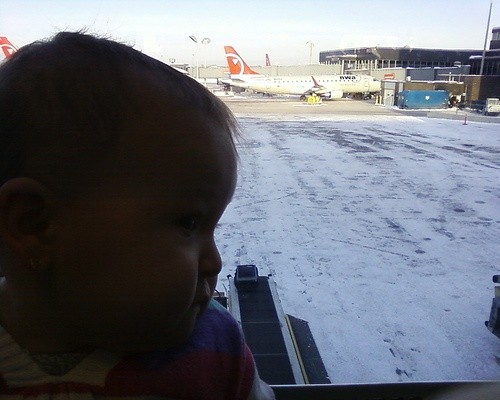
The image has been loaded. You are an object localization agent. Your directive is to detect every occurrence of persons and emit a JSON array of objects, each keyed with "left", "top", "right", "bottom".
[{"left": 0, "top": 32, "right": 276, "bottom": 400}]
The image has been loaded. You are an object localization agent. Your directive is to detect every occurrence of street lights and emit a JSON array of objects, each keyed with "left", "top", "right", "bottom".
[{"left": 189, "top": 34, "right": 199, "bottom": 79}]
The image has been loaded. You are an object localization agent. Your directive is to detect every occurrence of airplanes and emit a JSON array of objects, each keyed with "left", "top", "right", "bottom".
[{"left": 219, "top": 45, "right": 382, "bottom": 104}]
